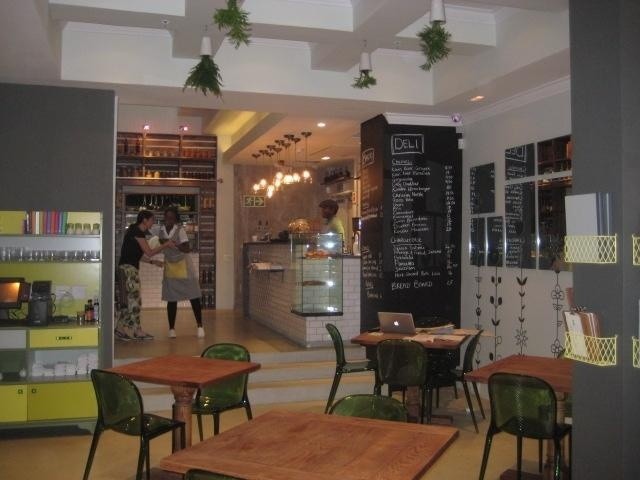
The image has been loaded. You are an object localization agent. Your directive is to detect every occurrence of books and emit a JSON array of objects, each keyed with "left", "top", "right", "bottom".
[
  {"left": 25, "top": 211, "right": 68, "bottom": 236},
  {"left": 564, "top": 306, "right": 600, "bottom": 360}
]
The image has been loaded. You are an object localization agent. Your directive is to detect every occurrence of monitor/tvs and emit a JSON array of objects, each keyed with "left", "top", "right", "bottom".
[{"left": 0, "top": 278, "right": 25, "bottom": 309}]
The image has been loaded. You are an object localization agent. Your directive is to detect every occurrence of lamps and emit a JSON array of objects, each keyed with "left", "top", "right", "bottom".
[
  {"left": 178, "top": 121, "right": 192, "bottom": 132},
  {"left": 248, "top": 129, "right": 315, "bottom": 200},
  {"left": 143, "top": 118, "right": 155, "bottom": 131}
]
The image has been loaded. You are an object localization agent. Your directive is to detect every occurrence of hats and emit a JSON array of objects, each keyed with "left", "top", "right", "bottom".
[{"left": 319, "top": 200, "right": 338, "bottom": 209}]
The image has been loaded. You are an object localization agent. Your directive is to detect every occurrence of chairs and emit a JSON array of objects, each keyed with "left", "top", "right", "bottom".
[
  {"left": 168, "top": 342, "right": 256, "bottom": 455},
  {"left": 79, "top": 365, "right": 188, "bottom": 480},
  {"left": 472, "top": 369, "right": 574, "bottom": 480},
  {"left": 320, "top": 319, "right": 489, "bottom": 434}
]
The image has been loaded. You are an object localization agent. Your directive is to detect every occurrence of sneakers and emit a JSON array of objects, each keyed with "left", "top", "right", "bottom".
[
  {"left": 167, "top": 329, "right": 176, "bottom": 338},
  {"left": 198, "top": 324, "right": 204, "bottom": 338},
  {"left": 115, "top": 328, "right": 132, "bottom": 342},
  {"left": 133, "top": 333, "right": 154, "bottom": 340}
]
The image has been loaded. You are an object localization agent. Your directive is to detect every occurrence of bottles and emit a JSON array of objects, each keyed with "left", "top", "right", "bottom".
[
  {"left": 85, "top": 295, "right": 100, "bottom": 323},
  {"left": 324, "top": 165, "right": 350, "bottom": 183},
  {"left": 200, "top": 188, "right": 216, "bottom": 308},
  {"left": 126, "top": 192, "right": 198, "bottom": 232},
  {"left": 66, "top": 223, "right": 101, "bottom": 236},
  {"left": 115, "top": 135, "right": 215, "bottom": 180}
]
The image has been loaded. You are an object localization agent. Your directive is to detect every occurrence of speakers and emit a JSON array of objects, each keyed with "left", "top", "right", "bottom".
[{"left": 28, "top": 297, "right": 52, "bottom": 326}]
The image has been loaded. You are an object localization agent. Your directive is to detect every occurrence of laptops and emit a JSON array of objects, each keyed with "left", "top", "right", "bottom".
[{"left": 377, "top": 311, "right": 418, "bottom": 335}]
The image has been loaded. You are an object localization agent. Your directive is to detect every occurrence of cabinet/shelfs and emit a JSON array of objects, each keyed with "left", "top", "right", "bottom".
[
  {"left": 1, "top": 226, "right": 100, "bottom": 430},
  {"left": 318, "top": 174, "right": 356, "bottom": 203},
  {"left": 291, "top": 248, "right": 339, "bottom": 293},
  {"left": 116, "top": 130, "right": 217, "bottom": 182}
]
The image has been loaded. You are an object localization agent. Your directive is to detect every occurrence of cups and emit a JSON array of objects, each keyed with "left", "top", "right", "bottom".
[
  {"left": 0, "top": 247, "right": 101, "bottom": 262},
  {"left": 251, "top": 236, "right": 258, "bottom": 243}
]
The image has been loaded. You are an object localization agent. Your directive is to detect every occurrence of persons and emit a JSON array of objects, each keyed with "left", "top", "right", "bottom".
[
  {"left": 319, "top": 199, "right": 347, "bottom": 251},
  {"left": 159, "top": 208, "right": 205, "bottom": 340},
  {"left": 114, "top": 210, "right": 154, "bottom": 344}
]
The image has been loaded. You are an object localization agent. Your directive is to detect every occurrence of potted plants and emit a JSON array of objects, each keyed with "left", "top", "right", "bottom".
[
  {"left": 417, "top": 0, "right": 456, "bottom": 72},
  {"left": 181, "top": 35, "right": 227, "bottom": 106},
  {"left": 349, "top": 49, "right": 378, "bottom": 92}
]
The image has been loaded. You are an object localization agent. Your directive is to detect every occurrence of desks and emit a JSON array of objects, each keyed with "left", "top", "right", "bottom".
[
  {"left": 155, "top": 404, "right": 464, "bottom": 480},
  {"left": 100, "top": 351, "right": 261, "bottom": 460},
  {"left": 461, "top": 352, "right": 573, "bottom": 479}
]
